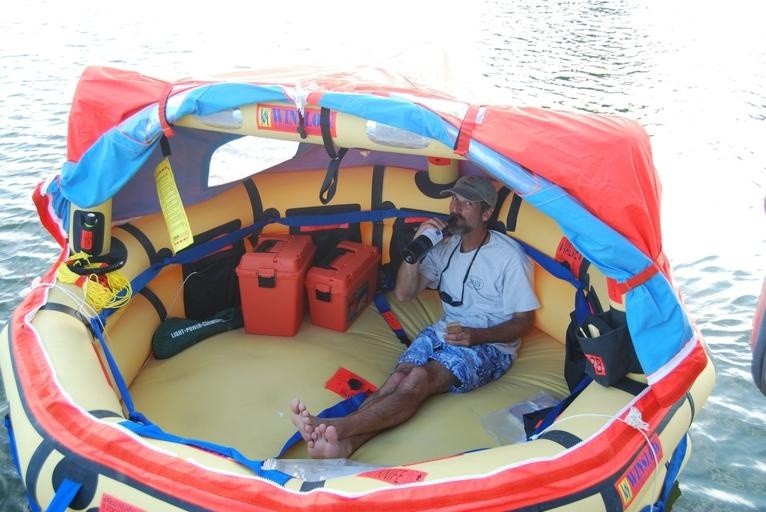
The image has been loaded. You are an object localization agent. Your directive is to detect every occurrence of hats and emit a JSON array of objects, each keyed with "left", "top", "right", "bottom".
[{"left": 439, "top": 175, "right": 498, "bottom": 209}]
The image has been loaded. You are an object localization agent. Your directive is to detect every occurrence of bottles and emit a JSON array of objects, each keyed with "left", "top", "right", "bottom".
[{"left": 401, "top": 216, "right": 458, "bottom": 264}]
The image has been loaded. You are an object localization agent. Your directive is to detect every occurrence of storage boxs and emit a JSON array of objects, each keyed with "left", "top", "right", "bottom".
[{"left": 237, "top": 232, "right": 382, "bottom": 336}]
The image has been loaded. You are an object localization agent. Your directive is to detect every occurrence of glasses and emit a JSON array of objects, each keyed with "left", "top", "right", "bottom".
[{"left": 437, "top": 278, "right": 465, "bottom": 306}]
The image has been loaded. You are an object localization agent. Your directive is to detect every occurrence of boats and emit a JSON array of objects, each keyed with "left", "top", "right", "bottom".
[{"left": 0, "top": 66, "right": 717, "bottom": 512}]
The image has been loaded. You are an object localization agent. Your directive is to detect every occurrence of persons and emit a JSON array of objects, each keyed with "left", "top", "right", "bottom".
[{"left": 290, "top": 175, "right": 541, "bottom": 459}]
